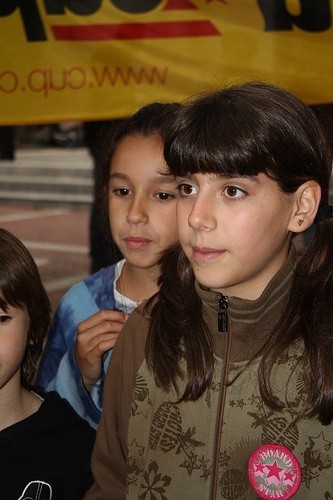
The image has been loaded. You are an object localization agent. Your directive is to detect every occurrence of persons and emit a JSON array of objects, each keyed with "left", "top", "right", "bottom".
[
  {"left": 0, "top": 227, "right": 96, "bottom": 500},
  {"left": 33, "top": 102, "right": 191, "bottom": 429},
  {"left": 83, "top": 81, "right": 333, "bottom": 500}
]
